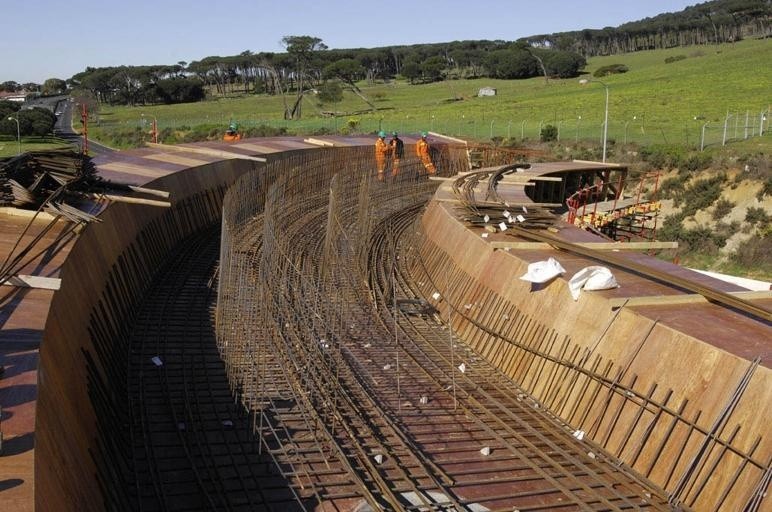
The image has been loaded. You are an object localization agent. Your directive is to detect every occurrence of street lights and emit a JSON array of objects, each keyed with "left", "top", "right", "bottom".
[
  {"left": 8, "top": 116, "right": 21, "bottom": 155},
  {"left": 141, "top": 112, "right": 158, "bottom": 143},
  {"left": 578, "top": 78, "right": 609, "bottom": 162}
]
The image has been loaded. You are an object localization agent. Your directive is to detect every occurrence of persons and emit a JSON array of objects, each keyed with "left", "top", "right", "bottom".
[
  {"left": 388, "top": 130, "right": 406, "bottom": 183},
  {"left": 374, "top": 130, "right": 395, "bottom": 183},
  {"left": 223, "top": 120, "right": 241, "bottom": 142},
  {"left": 415, "top": 131, "right": 440, "bottom": 175}
]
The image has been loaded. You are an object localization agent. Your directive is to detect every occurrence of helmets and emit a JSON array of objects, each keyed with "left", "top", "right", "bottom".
[
  {"left": 421, "top": 131, "right": 428, "bottom": 137},
  {"left": 378, "top": 130, "right": 386, "bottom": 137},
  {"left": 392, "top": 129, "right": 398, "bottom": 136},
  {"left": 229, "top": 123, "right": 238, "bottom": 129}
]
